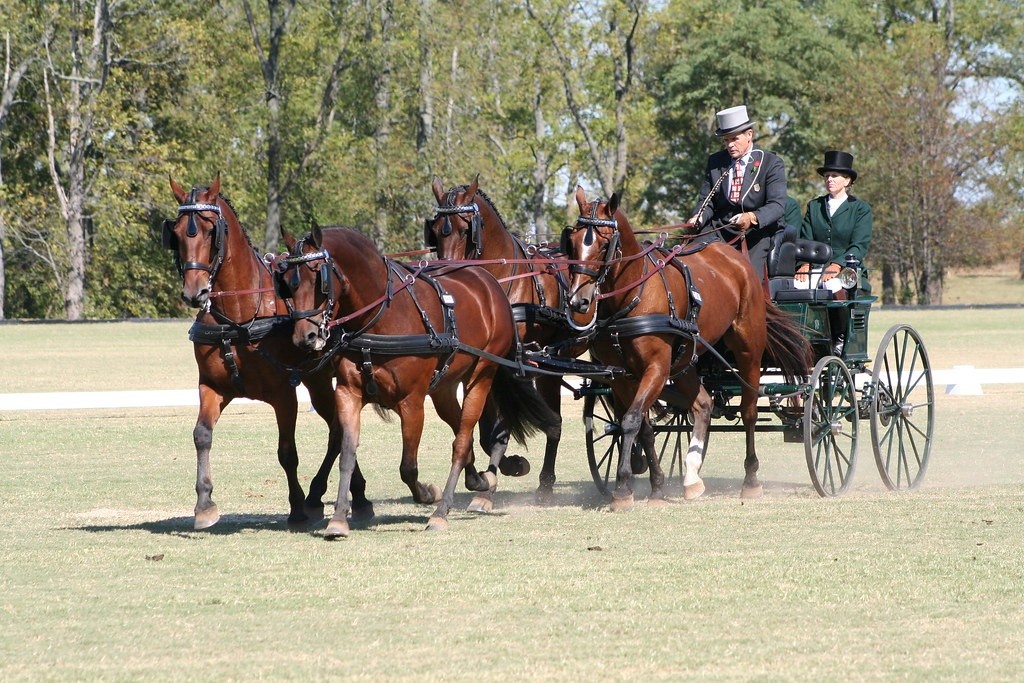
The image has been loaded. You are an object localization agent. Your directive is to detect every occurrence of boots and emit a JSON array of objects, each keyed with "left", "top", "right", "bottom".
[{"left": 831, "top": 289, "right": 848, "bottom": 357}]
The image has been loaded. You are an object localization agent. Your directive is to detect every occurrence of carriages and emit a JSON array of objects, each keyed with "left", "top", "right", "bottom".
[{"left": 163, "top": 168, "right": 934, "bottom": 539}]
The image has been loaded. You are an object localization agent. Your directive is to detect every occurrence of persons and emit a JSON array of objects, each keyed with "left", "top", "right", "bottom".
[
  {"left": 784, "top": 196, "right": 802, "bottom": 239},
  {"left": 794, "top": 151, "right": 873, "bottom": 356},
  {"left": 687, "top": 105, "right": 787, "bottom": 283}
]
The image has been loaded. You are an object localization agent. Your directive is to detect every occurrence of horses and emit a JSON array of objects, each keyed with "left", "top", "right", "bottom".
[
  {"left": 431, "top": 173, "right": 605, "bottom": 513},
  {"left": 279, "top": 221, "right": 562, "bottom": 540},
  {"left": 567, "top": 184, "right": 826, "bottom": 513},
  {"left": 168, "top": 170, "right": 375, "bottom": 530}
]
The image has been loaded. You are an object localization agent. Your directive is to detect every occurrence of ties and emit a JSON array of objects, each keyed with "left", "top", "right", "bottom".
[{"left": 730, "top": 160, "right": 744, "bottom": 204}]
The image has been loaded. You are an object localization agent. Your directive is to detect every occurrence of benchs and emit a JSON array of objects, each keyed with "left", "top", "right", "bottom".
[{"left": 767, "top": 226, "right": 870, "bottom": 339}]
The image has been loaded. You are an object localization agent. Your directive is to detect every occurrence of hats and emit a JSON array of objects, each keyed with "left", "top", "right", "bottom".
[
  {"left": 816, "top": 151, "right": 857, "bottom": 181},
  {"left": 714, "top": 105, "right": 756, "bottom": 137}
]
